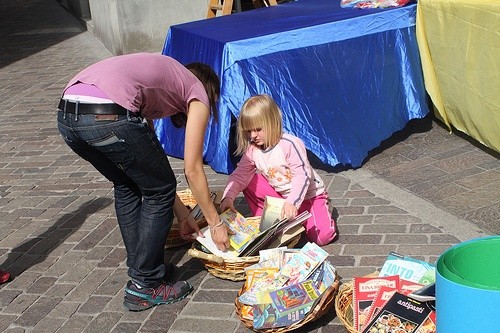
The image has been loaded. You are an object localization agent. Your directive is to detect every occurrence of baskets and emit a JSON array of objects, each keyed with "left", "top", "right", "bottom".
[
  {"left": 163, "top": 187, "right": 224, "bottom": 245},
  {"left": 233, "top": 268, "right": 340, "bottom": 333},
  {"left": 334, "top": 274, "right": 379, "bottom": 333},
  {"left": 188, "top": 216, "right": 303, "bottom": 280}
]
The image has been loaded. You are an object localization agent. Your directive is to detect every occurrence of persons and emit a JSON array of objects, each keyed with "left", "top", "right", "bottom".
[
  {"left": 219, "top": 94, "right": 337, "bottom": 246},
  {"left": 57, "top": 52, "right": 236, "bottom": 312}
]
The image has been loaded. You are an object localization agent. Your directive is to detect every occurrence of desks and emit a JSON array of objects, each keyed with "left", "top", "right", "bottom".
[{"left": 150, "top": 0, "right": 500, "bottom": 176}]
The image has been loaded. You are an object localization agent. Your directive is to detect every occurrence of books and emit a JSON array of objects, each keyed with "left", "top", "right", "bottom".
[{"left": 175, "top": 188, "right": 437, "bottom": 333}]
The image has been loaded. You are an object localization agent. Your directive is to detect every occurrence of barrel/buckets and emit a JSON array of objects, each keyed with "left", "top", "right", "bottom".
[{"left": 435, "top": 235, "right": 500, "bottom": 333}]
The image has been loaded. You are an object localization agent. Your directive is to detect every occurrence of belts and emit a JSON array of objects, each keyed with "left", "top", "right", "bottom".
[{"left": 58, "top": 97, "right": 138, "bottom": 116}]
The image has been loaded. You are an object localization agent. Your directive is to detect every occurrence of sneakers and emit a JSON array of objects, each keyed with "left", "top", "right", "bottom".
[
  {"left": 161, "top": 262, "right": 176, "bottom": 279},
  {"left": 124, "top": 280, "right": 191, "bottom": 310}
]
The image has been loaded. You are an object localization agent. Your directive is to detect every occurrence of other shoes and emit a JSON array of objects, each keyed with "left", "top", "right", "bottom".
[{"left": 0, "top": 269, "right": 11, "bottom": 283}]
